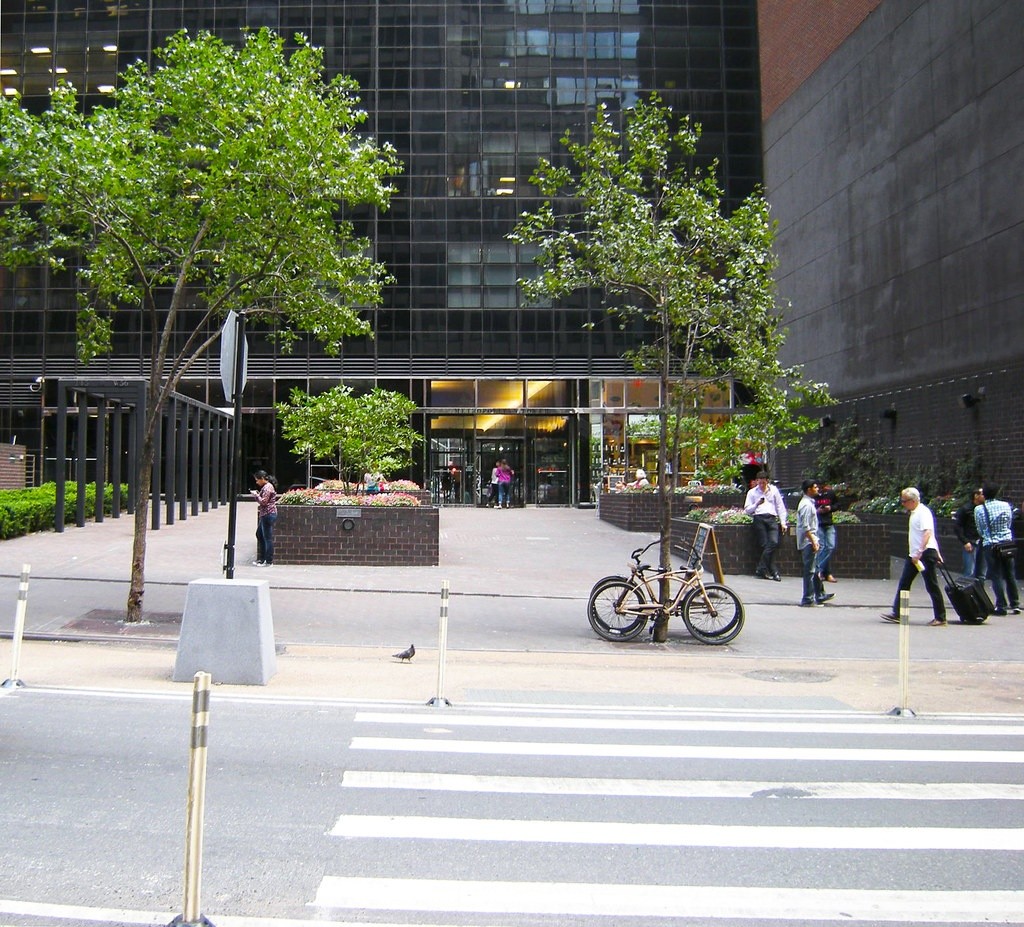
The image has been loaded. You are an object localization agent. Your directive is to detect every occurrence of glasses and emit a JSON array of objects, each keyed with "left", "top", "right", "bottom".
[{"left": 901, "top": 498, "right": 912, "bottom": 503}]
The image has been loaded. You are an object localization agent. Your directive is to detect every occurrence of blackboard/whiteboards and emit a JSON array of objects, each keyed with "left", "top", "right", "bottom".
[
  {"left": 608, "top": 476, "right": 625, "bottom": 494},
  {"left": 684, "top": 523, "right": 710, "bottom": 582}
]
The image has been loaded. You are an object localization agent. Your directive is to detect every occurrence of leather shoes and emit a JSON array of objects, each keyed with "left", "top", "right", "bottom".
[
  {"left": 773, "top": 572, "right": 781, "bottom": 581},
  {"left": 757, "top": 574, "right": 774, "bottom": 580}
]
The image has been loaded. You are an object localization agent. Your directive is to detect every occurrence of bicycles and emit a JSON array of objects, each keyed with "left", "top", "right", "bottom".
[{"left": 586, "top": 538, "right": 746, "bottom": 646}]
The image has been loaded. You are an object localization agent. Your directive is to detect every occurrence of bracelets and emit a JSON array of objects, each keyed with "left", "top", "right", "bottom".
[{"left": 916, "top": 549, "right": 923, "bottom": 554}]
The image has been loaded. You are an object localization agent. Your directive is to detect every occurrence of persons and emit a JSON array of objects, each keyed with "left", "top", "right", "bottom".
[
  {"left": 880, "top": 487, "right": 948, "bottom": 627},
  {"left": 486, "top": 459, "right": 514, "bottom": 509},
  {"left": 364, "top": 465, "right": 387, "bottom": 495},
  {"left": 744, "top": 471, "right": 787, "bottom": 582},
  {"left": 814, "top": 478, "right": 837, "bottom": 583},
  {"left": 250, "top": 470, "right": 278, "bottom": 567},
  {"left": 973, "top": 483, "right": 1022, "bottom": 614},
  {"left": 796, "top": 480, "right": 836, "bottom": 607},
  {"left": 955, "top": 486, "right": 988, "bottom": 579},
  {"left": 628, "top": 469, "right": 650, "bottom": 486}
]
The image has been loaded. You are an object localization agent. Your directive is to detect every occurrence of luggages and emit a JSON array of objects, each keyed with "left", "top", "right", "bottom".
[{"left": 937, "top": 561, "right": 995, "bottom": 624}]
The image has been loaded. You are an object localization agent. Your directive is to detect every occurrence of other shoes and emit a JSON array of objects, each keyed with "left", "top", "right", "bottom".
[
  {"left": 252, "top": 560, "right": 273, "bottom": 567},
  {"left": 1013, "top": 607, "right": 1021, "bottom": 614},
  {"left": 927, "top": 619, "right": 948, "bottom": 627},
  {"left": 506, "top": 503, "right": 510, "bottom": 508},
  {"left": 486, "top": 503, "right": 491, "bottom": 508},
  {"left": 494, "top": 505, "right": 502, "bottom": 509},
  {"left": 802, "top": 593, "right": 836, "bottom": 607},
  {"left": 819, "top": 574, "right": 837, "bottom": 582},
  {"left": 993, "top": 606, "right": 1008, "bottom": 616},
  {"left": 879, "top": 612, "right": 900, "bottom": 623}
]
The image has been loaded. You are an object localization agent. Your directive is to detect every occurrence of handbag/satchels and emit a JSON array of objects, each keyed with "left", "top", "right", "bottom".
[
  {"left": 993, "top": 541, "right": 1017, "bottom": 559},
  {"left": 367, "top": 481, "right": 389, "bottom": 491}
]
